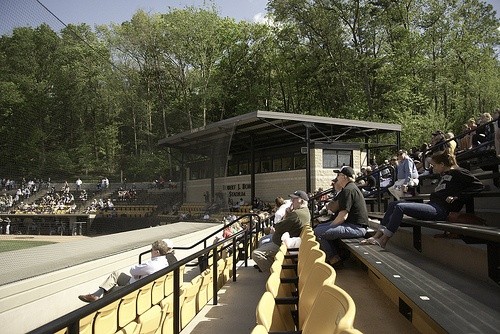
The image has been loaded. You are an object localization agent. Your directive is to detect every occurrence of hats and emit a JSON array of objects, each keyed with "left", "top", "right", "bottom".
[
  {"left": 331, "top": 177, "right": 337, "bottom": 182},
  {"left": 396, "top": 149, "right": 406, "bottom": 153},
  {"left": 333, "top": 166, "right": 355, "bottom": 179},
  {"left": 288, "top": 190, "right": 309, "bottom": 201}
]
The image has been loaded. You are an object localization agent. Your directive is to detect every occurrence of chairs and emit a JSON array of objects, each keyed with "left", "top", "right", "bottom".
[{"left": 7, "top": 203, "right": 364, "bottom": 334}]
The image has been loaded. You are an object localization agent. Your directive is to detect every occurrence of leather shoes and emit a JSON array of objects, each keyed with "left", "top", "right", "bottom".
[{"left": 78, "top": 295, "right": 100, "bottom": 302}]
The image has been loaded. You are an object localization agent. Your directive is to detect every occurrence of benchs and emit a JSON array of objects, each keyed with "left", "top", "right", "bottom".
[
  {"left": 316, "top": 150, "right": 500, "bottom": 334},
  {"left": 0, "top": 186, "right": 184, "bottom": 204}
]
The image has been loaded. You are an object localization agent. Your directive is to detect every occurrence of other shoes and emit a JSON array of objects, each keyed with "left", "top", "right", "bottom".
[
  {"left": 327, "top": 259, "right": 344, "bottom": 268},
  {"left": 368, "top": 238, "right": 380, "bottom": 246},
  {"left": 360, "top": 237, "right": 373, "bottom": 244}
]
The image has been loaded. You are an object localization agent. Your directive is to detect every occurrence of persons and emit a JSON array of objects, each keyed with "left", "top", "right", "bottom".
[
  {"left": 0, "top": 173, "right": 165, "bottom": 235},
  {"left": 78, "top": 241, "right": 169, "bottom": 304},
  {"left": 161, "top": 239, "right": 178, "bottom": 266},
  {"left": 167, "top": 112, "right": 500, "bottom": 277}
]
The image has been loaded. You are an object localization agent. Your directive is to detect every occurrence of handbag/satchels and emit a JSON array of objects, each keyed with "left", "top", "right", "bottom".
[{"left": 448, "top": 212, "right": 489, "bottom": 226}]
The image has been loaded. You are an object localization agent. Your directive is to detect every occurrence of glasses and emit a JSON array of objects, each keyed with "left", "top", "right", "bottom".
[{"left": 396, "top": 152, "right": 406, "bottom": 156}]
